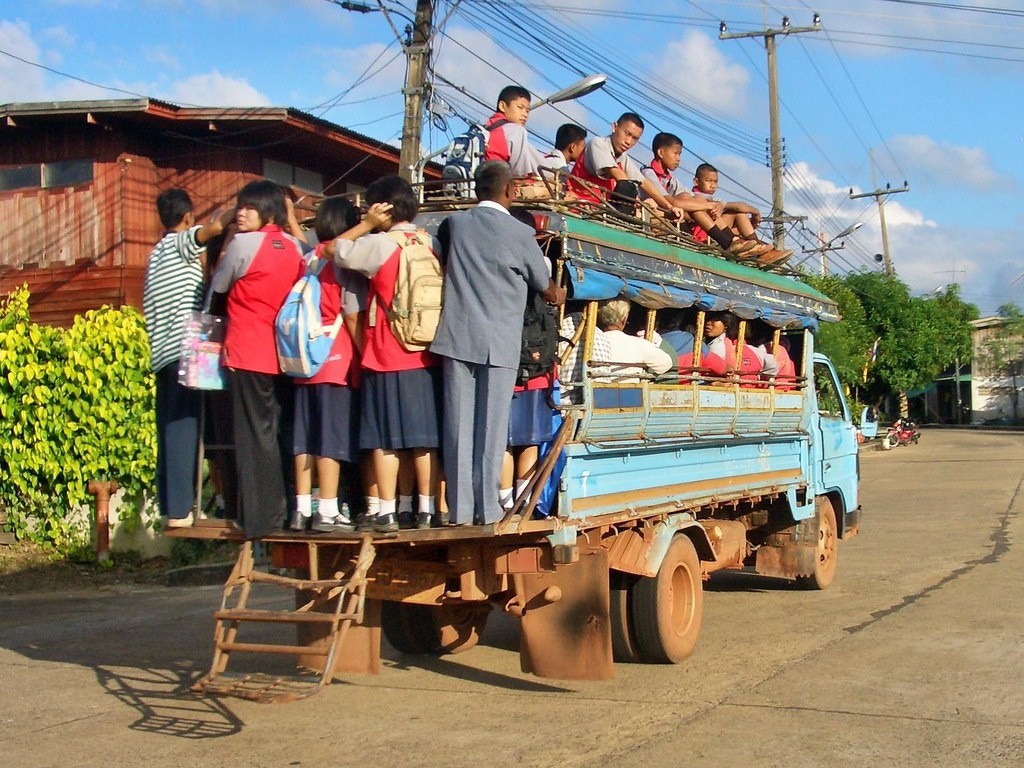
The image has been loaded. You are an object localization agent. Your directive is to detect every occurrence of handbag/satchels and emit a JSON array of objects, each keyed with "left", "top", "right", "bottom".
[
  {"left": 177, "top": 279, "right": 228, "bottom": 391},
  {"left": 606, "top": 179, "right": 642, "bottom": 216}
]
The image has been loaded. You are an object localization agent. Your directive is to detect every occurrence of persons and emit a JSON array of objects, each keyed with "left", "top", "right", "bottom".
[
  {"left": 429, "top": 159, "right": 565, "bottom": 525},
  {"left": 143, "top": 85, "right": 796, "bottom": 541}
]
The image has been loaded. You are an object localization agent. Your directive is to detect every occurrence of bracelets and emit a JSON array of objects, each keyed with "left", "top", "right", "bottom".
[{"left": 550, "top": 292, "right": 558, "bottom": 305}]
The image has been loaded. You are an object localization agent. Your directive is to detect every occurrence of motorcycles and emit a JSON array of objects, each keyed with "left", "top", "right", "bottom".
[{"left": 883, "top": 416, "right": 922, "bottom": 449}]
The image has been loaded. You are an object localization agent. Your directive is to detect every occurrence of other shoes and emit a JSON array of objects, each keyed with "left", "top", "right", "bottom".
[
  {"left": 289, "top": 510, "right": 313, "bottom": 531},
  {"left": 311, "top": 511, "right": 357, "bottom": 532},
  {"left": 161, "top": 511, "right": 208, "bottom": 528},
  {"left": 357, "top": 510, "right": 450, "bottom": 532},
  {"left": 504, "top": 501, "right": 540, "bottom": 521}
]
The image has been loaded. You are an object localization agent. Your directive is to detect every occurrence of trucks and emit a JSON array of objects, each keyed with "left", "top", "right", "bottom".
[{"left": 159, "top": 165, "right": 879, "bottom": 700}]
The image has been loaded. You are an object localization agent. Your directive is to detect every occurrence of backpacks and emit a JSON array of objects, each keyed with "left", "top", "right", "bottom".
[
  {"left": 515, "top": 285, "right": 575, "bottom": 410},
  {"left": 274, "top": 248, "right": 345, "bottom": 378},
  {"left": 368, "top": 227, "right": 445, "bottom": 351},
  {"left": 441, "top": 119, "right": 512, "bottom": 198}
]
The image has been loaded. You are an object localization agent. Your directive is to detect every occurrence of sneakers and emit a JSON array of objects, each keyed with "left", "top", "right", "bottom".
[
  {"left": 722, "top": 238, "right": 757, "bottom": 254},
  {"left": 736, "top": 243, "right": 774, "bottom": 259},
  {"left": 755, "top": 249, "right": 793, "bottom": 272}
]
